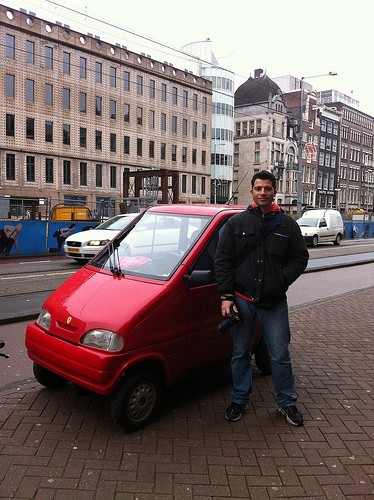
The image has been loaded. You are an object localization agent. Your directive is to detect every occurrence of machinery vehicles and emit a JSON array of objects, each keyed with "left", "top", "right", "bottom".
[{"left": 49, "top": 202, "right": 93, "bottom": 220}]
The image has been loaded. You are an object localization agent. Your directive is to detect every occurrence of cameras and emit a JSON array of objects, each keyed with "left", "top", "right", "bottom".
[{"left": 216, "top": 304, "right": 244, "bottom": 335}]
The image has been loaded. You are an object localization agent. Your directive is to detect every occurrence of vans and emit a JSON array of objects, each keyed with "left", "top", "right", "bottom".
[
  {"left": 296, "top": 208, "right": 345, "bottom": 248},
  {"left": 295, "top": 72, "right": 340, "bottom": 222}
]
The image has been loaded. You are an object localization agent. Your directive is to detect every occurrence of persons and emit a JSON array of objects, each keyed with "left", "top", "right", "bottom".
[
  {"left": 48, "top": 224, "right": 74, "bottom": 253},
  {"left": 214, "top": 170, "right": 309, "bottom": 426},
  {"left": 1, "top": 222, "right": 22, "bottom": 255},
  {"left": 351, "top": 224, "right": 370, "bottom": 238}
]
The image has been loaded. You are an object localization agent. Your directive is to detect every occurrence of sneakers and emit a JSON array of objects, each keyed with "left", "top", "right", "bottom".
[
  {"left": 225, "top": 401, "right": 248, "bottom": 421},
  {"left": 276, "top": 402, "right": 304, "bottom": 426}
]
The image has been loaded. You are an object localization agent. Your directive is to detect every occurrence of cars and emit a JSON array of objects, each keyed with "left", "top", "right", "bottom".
[
  {"left": 23, "top": 203, "right": 273, "bottom": 435},
  {"left": 62, "top": 211, "right": 201, "bottom": 266}
]
